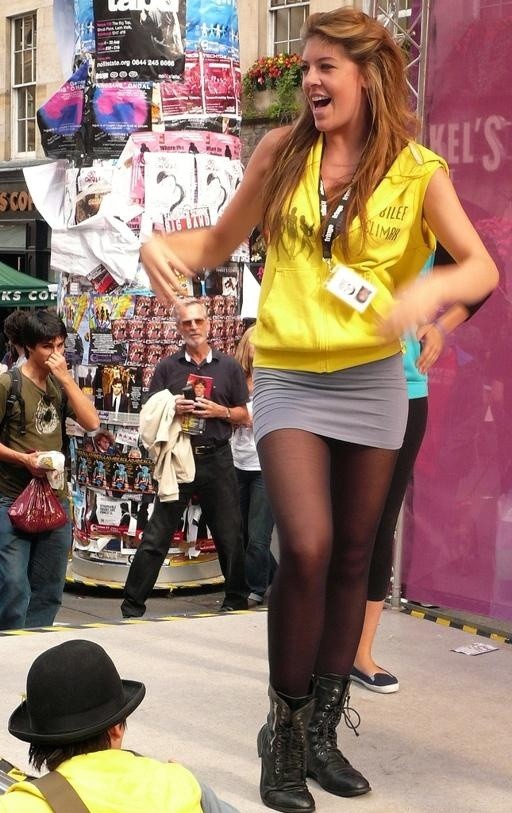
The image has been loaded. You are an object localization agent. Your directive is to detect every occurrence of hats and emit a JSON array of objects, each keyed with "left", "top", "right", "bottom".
[{"left": 8, "top": 640, "right": 147, "bottom": 746}]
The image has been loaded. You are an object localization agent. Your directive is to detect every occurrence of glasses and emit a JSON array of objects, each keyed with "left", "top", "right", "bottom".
[
  {"left": 179, "top": 318, "right": 209, "bottom": 327},
  {"left": 42, "top": 394, "right": 51, "bottom": 423}
]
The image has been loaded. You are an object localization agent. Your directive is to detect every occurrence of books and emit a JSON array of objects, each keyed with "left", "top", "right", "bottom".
[{"left": 177, "top": 373, "right": 213, "bottom": 437}]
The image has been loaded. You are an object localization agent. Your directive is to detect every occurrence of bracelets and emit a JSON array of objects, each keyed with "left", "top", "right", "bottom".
[{"left": 221, "top": 405, "right": 234, "bottom": 423}]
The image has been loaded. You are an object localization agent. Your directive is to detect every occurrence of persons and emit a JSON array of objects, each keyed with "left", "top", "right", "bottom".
[
  {"left": 102, "top": 377, "right": 129, "bottom": 413},
  {"left": 0, "top": 639, "right": 233, "bottom": 811},
  {"left": 2, "top": 308, "right": 34, "bottom": 367},
  {"left": 0, "top": 310, "right": 103, "bottom": 630},
  {"left": 76, "top": 429, "right": 153, "bottom": 492},
  {"left": 120, "top": 297, "right": 251, "bottom": 620},
  {"left": 137, "top": 2, "right": 502, "bottom": 812},
  {"left": 348, "top": 230, "right": 494, "bottom": 696},
  {"left": 355, "top": 286, "right": 373, "bottom": 305},
  {"left": 228, "top": 320, "right": 280, "bottom": 610},
  {"left": 183, "top": 377, "right": 209, "bottom": 418}
]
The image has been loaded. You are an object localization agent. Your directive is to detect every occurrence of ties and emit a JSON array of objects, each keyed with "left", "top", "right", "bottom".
[{"left": 113, "top": 398, "right": 116, "bottom": 418}]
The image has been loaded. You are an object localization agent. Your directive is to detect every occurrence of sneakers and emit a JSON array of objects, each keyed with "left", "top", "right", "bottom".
[{"left": 348, "top": 663, "right": 401, "bottom": 694}]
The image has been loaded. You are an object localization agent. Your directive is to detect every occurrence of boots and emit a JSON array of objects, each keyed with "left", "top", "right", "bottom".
[
  {"left": 257, "top": 681, "right": 315, "bottom": 813},
  {"left": 306, "top": 672, "right": 373, "bottom": 799}
]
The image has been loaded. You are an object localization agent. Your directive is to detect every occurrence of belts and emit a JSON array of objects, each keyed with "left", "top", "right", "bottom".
[{"left": 191, "top": 438, "right": 228, "bottom": 454}]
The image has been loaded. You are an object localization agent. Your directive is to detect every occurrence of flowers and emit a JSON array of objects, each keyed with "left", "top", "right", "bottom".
[{"left": 248, "top": 53, "right": 302, "bottom": 91}]
